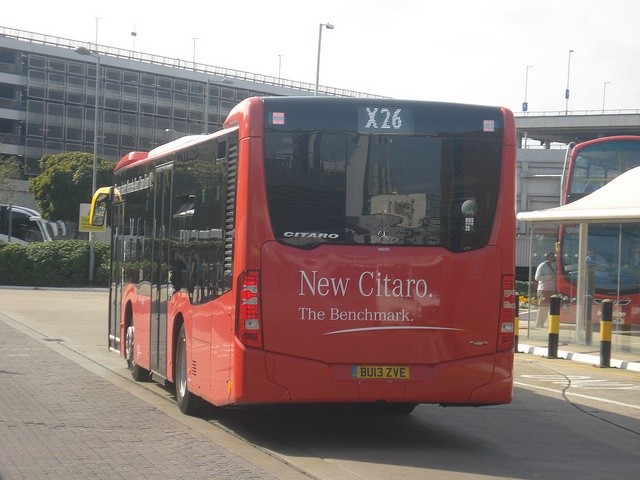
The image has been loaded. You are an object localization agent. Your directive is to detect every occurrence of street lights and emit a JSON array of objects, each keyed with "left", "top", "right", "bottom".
[
  {"left": 315, "top": 21, "right": 335, "bottom": 92},
  {"left": 73, "top": 46, "right": 100, "bottom": 198}
]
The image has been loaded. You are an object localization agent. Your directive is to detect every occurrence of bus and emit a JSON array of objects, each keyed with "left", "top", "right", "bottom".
[
  {"left": 555, "top": 135, "right": 640, "bottom": 326},
  {"left": 85, "top": 96, "right": 517, "bottom": 416},
  {"left": 1, "top": 204, "right": 66, "bottom": 246}
]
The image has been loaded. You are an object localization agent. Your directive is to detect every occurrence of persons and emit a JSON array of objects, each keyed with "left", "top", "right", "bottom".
[
  {"left": 533, "top": 251, "right": 557, "bottom": 327},
  {"left": 587, "top": 248, "right": 606, "bottom": 263}
]
